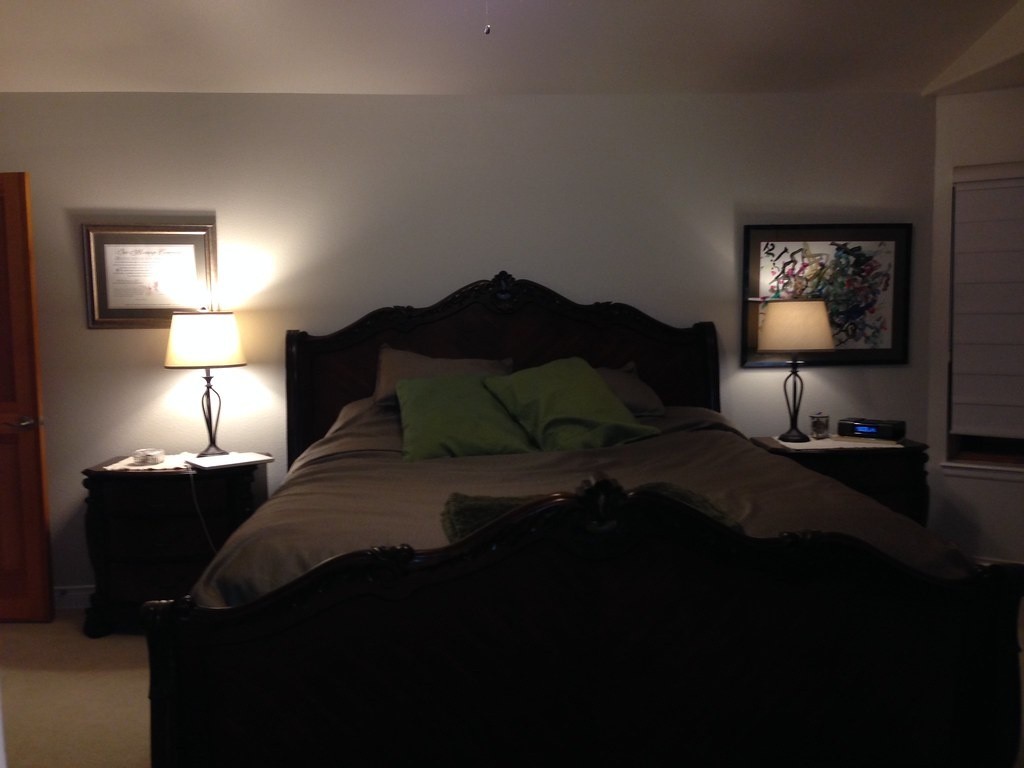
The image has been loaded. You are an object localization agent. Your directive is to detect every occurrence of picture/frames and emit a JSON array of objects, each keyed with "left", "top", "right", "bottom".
[
  {"left": 80, "top": 222, "right": 216, "bottom": 331},
  {"left": 742, "top": 225, "right": 913, "bottom": 368}
]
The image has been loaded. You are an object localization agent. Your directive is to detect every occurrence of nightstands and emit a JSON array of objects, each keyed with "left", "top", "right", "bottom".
[
  {"left": 81, "top": 458, "right": 270, "bottom": 639},
  {"left": 753, "top": 436, "right": 932, "bottom": 527}
]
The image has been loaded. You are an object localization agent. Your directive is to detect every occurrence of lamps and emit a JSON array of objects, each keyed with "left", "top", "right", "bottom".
[
  {"left": 163, "top": 311, "right": 248, "bottom": 456},
  {"left": 760, "top": 302, "right": 834, "bottom": 444}
]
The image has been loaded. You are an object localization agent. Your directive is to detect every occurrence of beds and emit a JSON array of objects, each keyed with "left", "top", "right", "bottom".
[{"left": 149, "top": 272, "right": 1024, "bottom": 768}]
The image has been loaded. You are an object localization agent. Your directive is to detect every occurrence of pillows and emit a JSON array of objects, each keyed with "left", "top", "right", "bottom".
[
  {"left": 397, "top": 377, "right": 526, "bottom": 460},
  {"left": 373, "top": 349, "right": 506, "bottom": 410},
  {"left": 595, "top": 367, "right": 665, "bottom": 416},
  {"left": 485, "top": 359, "right": 657, "bottom": 449}
]
